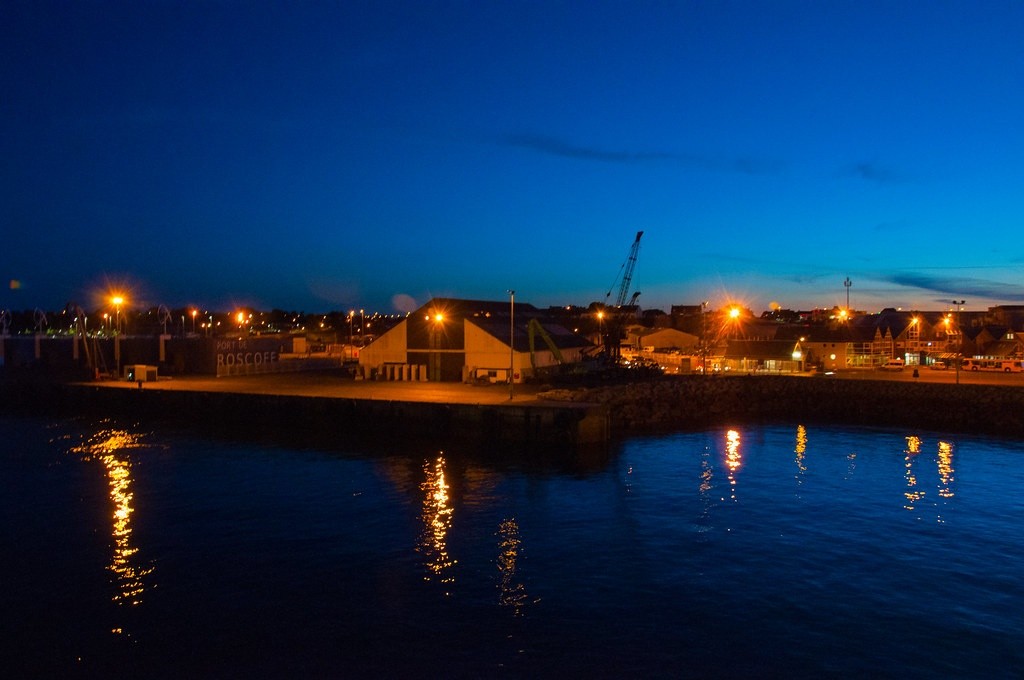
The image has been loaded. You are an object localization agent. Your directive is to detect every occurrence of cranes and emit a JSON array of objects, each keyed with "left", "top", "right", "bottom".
[{"left": 598, "top": 229, "right": 643, "bottom": 368}]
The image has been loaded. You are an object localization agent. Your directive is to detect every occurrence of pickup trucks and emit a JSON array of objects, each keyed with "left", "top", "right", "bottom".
[{"left": 804, "top": 363, "right": 817, "bottom": 371}]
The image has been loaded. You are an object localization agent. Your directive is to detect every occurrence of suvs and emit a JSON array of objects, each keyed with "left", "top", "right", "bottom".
[{"left": 345, "top": 364, "right": 359, "bottom": 376}]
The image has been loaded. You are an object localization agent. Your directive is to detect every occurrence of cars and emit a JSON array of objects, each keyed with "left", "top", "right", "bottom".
[
  {"left": 931, "top": 361, "right": 946, "bottom": 370},
  {"left": 618, "top": 357, "right": 659, "bottom": 370},
  {"left": 706, "top": 365, "right": 719, "bottom": 374}
]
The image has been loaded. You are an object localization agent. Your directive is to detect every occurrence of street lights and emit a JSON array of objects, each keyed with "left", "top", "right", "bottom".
[
  {"left": 830, "top": 354, "right": 836, "bottom": 372},
  {"left": 952, "top": 301, "right": 965, "bottom": 383},
  {"left": 597, "top": 311, "right": 604, "bottom": 345},
  {"left": 730, "top": 309, "right": 739, "bottom": 363},
  {"left": 913, "top": 318, "right": 917, "bottom": 369},
  {"left": 360, "top": 310, "right": 364, "bottom": 347},
  {"left": 191, "top": 309, "right": 197, "bottom": 333},
  {"left": 702, "top": 302, "right": 707, "bottom": 377},
  {"left": 350, "top": 310, "right": 355, "bottom": 364},
  {"left": 107, "top": 293, "right": 127, "bottom": 376},
  {"left": 506, "top": 290, "right": 516, "bottom": 399},
  {"left": 182, "top": 316, "right": 184, "bottom": 338}
]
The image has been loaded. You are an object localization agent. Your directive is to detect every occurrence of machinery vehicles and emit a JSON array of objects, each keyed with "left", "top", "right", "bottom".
[{"left": 528, "top": 318, "right": 591, "bottom": 387}]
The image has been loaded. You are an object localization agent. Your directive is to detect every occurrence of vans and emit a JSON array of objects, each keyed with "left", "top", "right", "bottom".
[{"left": 882, "top": 360, "right": 906, "bottom": 372}]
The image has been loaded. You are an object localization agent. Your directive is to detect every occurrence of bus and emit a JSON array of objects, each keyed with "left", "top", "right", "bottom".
[{"left": 961, "top": 358, "right": 1024, "bottom": 373}]
